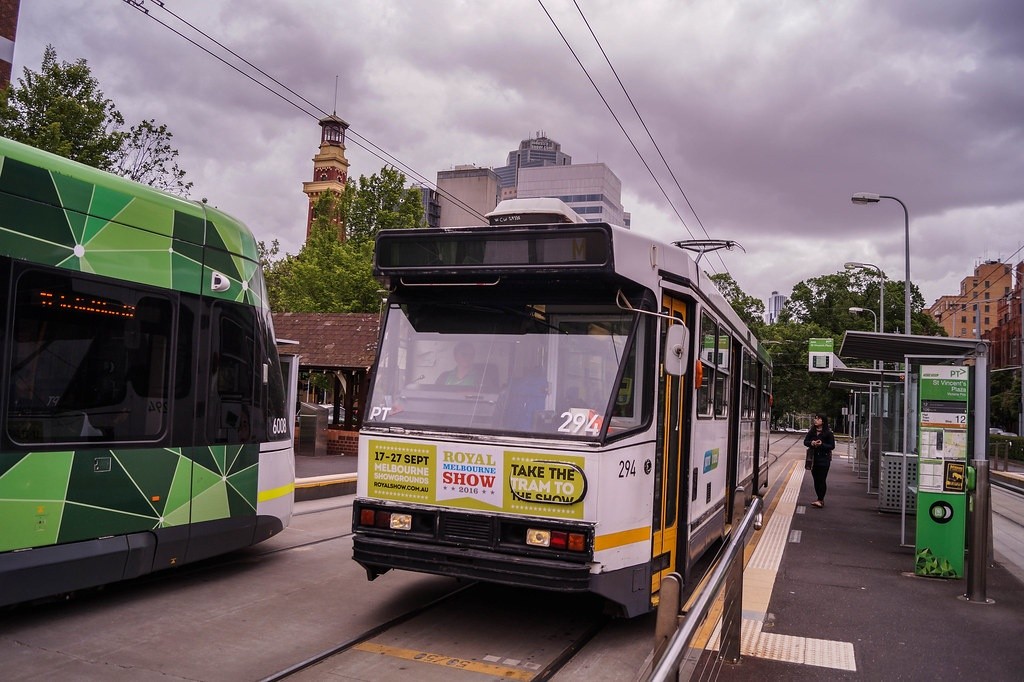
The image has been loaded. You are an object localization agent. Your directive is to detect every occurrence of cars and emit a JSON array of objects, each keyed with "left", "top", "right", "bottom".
[
  {"left": 294, "top": 404, "right": 345, "bottom": 424},
  {"left": 780, "top": 428, "right": 797, "bottom": 432},
  {"left": 989, "top": 428, "right": 1017, "bottom": 436},
  {"left": 797, "top": 429, "right": 808, "bottom": 433}
]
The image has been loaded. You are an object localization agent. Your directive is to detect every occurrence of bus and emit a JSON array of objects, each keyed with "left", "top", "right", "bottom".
[
  {"left": 350, "top": 197, "right": 771, "bottom": 617},
  {"left": 0, "top": 136, "right": 300, "bottom": 605}
]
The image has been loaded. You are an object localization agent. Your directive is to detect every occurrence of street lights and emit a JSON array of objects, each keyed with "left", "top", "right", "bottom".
[
  {"left": 851, "top": 191, "right": 911, "bottom": 365},
  {"left": 844, "top": 262, "right": 884, "bottom": 371},
  {"left": 848, "top": 307, "right": 877, "bottom": 370}
]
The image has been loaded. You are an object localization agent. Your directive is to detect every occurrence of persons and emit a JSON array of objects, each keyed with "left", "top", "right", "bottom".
[
  {"left": 435, "top": 341, "right": 490, "bottom": 390},
  {"left": 567, "top": 384, "right": 589, "bottom": 410},
  {"left": 803, "top": 413, "right": 835, "bottom": 507}
]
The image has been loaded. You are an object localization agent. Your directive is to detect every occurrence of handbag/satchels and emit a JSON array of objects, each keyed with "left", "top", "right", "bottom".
[{"left": 805, "top": 449, "right": 814, "bottom": 469}]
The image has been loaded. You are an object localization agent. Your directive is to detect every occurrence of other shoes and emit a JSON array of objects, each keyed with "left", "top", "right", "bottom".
[{"left": 811, "top": 500, "right": 824, "bottom": 507}]
